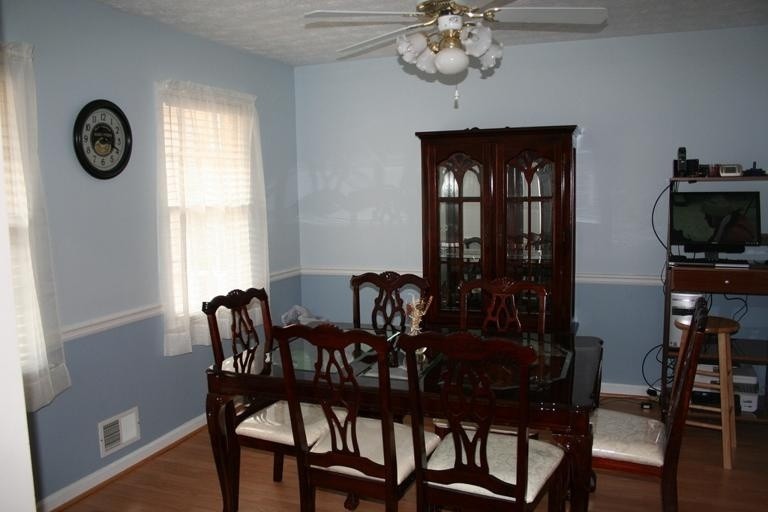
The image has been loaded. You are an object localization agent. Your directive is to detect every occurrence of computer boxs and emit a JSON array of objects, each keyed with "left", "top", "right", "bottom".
[{"left": 669, "top": 291, "right": 705, "bottom": 350}]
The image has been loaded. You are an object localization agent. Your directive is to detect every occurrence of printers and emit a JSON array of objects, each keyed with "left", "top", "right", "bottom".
[{"left": 689, "top": 362, "right": 761, "bottom": 414}]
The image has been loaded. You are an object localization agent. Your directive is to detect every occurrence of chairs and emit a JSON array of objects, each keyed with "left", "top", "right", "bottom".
[
  {"left": 341, "top": 270, "right": 430, "bottom": 508},
  {"left": 396, "top": 332, "right": 572, "bottom": 511},
  {"left": 269, "top": 323, "right": 441, "bottom": 511},
  {"left": 432, "top": 278, "right": 548, "bottom": 439},
  {"left": 202, "top": 286, "right": 350, "bottom": 511},
  {"left": 588, "top": 296, "right": 709, "bottom": 510}
]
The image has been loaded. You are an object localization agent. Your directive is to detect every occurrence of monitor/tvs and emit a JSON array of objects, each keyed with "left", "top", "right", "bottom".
[{"left": 669, "top": 190, "right": 761, "bottom": 260}]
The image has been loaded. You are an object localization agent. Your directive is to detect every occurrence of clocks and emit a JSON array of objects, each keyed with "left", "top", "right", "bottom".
[{"left": 73, "top": 98, "right": 134, "bottom": 180}]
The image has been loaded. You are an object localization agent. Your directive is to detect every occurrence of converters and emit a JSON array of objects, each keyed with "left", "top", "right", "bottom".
[
  {"left": 645, "top": 388, "right": 657, "bottom": 397},
  {"left": 641, "top": 401, "right": 651, "bottom": 410}
]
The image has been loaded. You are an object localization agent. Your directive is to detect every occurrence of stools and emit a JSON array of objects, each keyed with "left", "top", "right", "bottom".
[{"left": 667, "top": 316, "right": 739, "bottom": 471}]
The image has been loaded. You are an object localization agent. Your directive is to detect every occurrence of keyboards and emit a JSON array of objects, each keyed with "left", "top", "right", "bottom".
[{"left": 667, "top": 255, "right": 750, "bottom": 267}]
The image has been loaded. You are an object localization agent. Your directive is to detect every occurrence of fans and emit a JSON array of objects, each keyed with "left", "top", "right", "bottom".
[{"left": 302, "top": 0, "right": 609, "bottom": 58}]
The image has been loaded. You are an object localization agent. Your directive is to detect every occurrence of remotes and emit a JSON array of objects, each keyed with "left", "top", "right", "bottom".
[{"left": 714, "top": 263, "right": 749, "bottom": 268}]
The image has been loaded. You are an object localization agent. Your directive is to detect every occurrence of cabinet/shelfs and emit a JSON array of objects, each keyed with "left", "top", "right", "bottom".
[
  {"left": 660, "top": 261, "right": 768, "bottom": 425},
  {"left": 415, "top": 124, "right": 584, "bottom": 336}
]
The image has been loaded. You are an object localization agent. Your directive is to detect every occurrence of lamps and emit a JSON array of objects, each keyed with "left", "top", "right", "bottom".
[{"left": 395, "top": 13, "right": 507, "bottom": 78}]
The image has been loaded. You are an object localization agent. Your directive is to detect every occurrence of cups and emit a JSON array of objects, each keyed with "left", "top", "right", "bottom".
[{"left": 439, "top": 242, "right": 460, "bottom": 257}]
